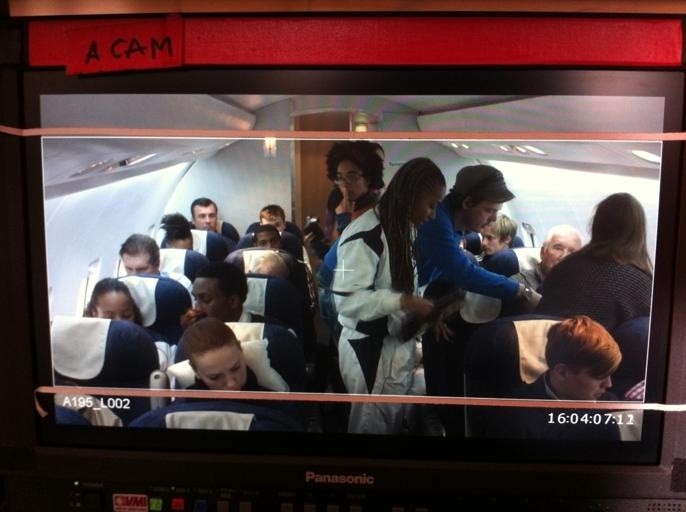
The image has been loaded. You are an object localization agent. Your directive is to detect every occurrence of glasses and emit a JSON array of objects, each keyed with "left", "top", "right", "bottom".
[{"left": 330, "top": 171, "right": 363, "bottom": 188}]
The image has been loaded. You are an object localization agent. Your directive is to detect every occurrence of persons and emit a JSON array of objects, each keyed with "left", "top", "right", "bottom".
[
  {"left": 257, "top": 205, "right": 287, "bottom": 233},
  {"left": 248, "top": 225, "right": 281, "bottom": 248},
  {"left": 189, "top": 262, "right": 278, "bottom": 323},
  {"left": 161, "top": 213, "right": 193, "bottom": 249},
  {"left": 313, "top": 141, "right": 654, "bottom": 444},
  {"left": 174, "top": 307, "right": 299, "bottom": 421},
  {"left": 120, "top": 233, "right": 161, "bottom": 274},
  {"left": 82, "top": 278, "right": 171, "bottom": 342},
  {"left": 188, "top": 198, "right": 218, "bottom": 233}
]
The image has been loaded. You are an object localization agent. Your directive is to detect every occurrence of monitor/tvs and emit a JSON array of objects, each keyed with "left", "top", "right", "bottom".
[{"left": 21, "top": 63, "right": 684, "bottom": 500}]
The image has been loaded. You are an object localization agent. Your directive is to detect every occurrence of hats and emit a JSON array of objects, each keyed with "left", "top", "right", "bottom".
[{"left": 447, "top": 164, "right": 516, "bottom": 205}]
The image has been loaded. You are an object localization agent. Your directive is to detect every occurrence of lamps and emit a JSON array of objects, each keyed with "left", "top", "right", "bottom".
[{"left": 261, "top": 138, "right": 278, "bottom": 160}]
